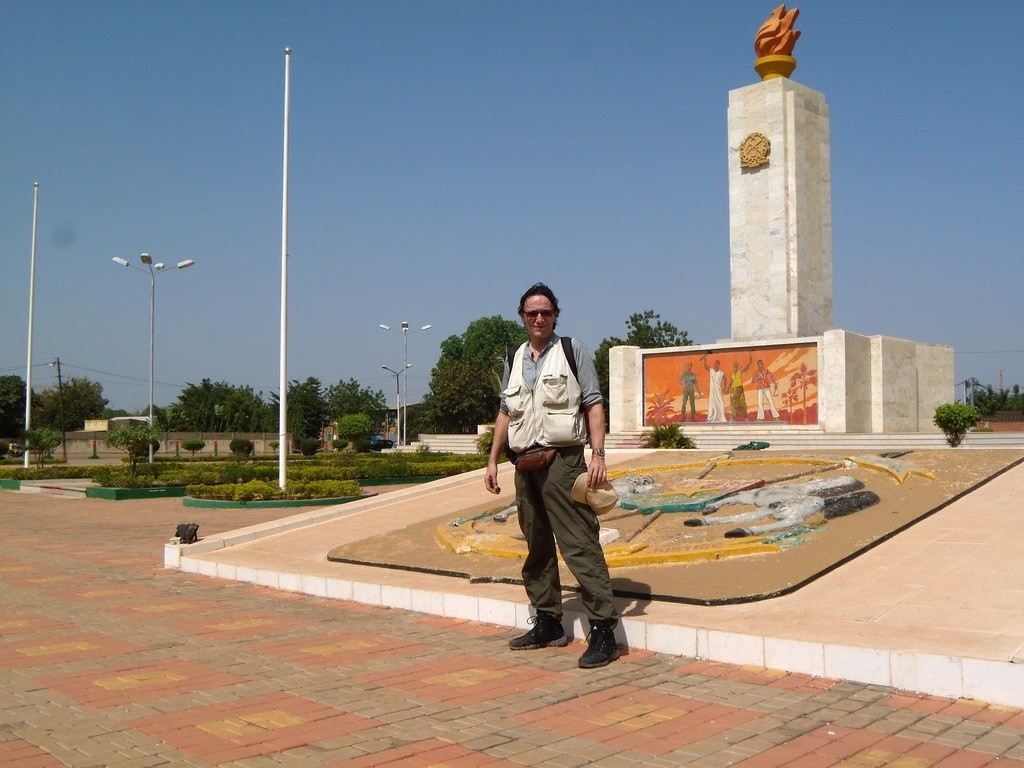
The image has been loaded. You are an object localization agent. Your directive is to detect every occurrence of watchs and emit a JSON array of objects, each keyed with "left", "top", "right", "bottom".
[{"left": 593, "top": 448, "right": 605, "bottom": 456}]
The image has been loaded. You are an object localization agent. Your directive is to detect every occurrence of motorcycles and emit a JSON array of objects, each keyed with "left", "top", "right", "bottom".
[{"left": 7, "top": 446, "right": 24, "bottom": 457}]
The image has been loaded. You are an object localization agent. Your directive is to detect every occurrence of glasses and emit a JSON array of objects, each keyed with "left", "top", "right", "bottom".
[{"left": 522, "top": 309, "right": 555, "bottom": 317}]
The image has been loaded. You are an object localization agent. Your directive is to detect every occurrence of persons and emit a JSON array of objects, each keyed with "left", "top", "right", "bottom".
[
  {"left": 484, "top": 285, "right": 619, "bottom": 668},
  {"left": 9, "top": 441, "right": 17, "bottom": 450}
]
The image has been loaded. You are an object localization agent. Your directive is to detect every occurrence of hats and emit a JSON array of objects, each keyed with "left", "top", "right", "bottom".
[{"left": 571, "top": 471, "right": 619, "bottom": 516}]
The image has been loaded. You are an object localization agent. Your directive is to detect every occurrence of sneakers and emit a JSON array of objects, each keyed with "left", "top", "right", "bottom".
[
  {"left": 508, "top": 613, "right": 568, "bottom": 650},
  {"left": 578, "top": 623, "right": 620, "bottom": 668}
]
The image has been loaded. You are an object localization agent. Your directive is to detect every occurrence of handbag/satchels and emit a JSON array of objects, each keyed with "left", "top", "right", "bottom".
[{"left": 513, "top": 446, "right": 558, "bottom": 472}]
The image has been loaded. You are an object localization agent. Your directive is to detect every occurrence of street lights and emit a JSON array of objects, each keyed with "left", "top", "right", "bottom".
[
  {"left": 380, "top": 321, "right": 433, "bottom": 447},
  {"left": 112, "top": 252, "right": 195, "bottom": 463},
  {"left": 381, "top": 364, "right": 412, "bottom": 446}
]
